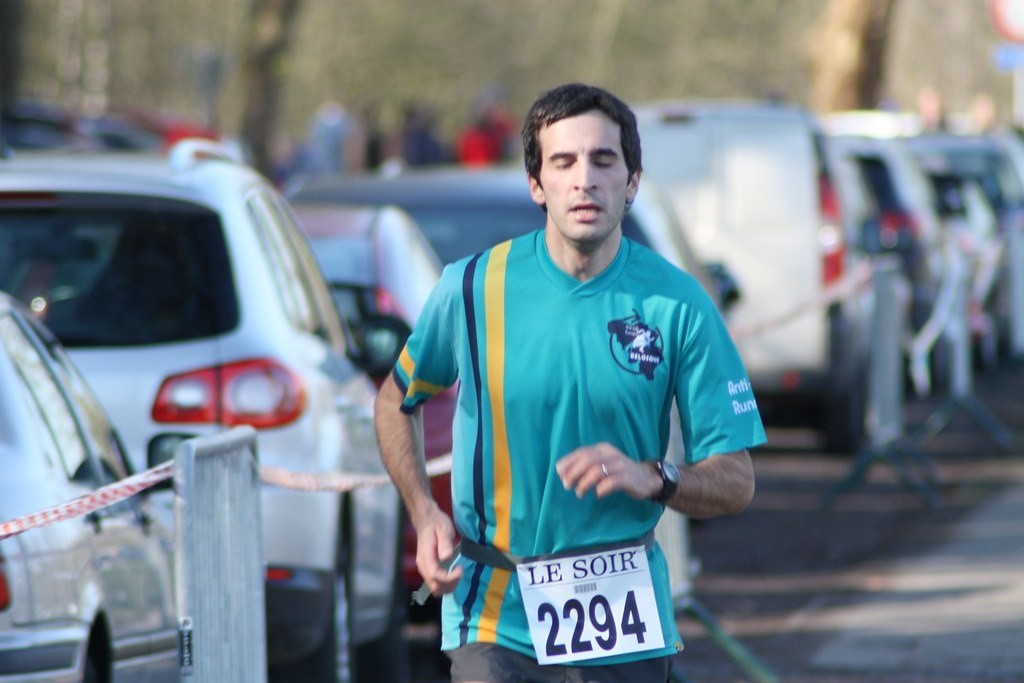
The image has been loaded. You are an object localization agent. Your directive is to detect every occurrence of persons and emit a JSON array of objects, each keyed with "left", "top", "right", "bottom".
[
  {"left": 301, "top": 98, "right": 519, "bottom": 177},
  {"left": 374, "top": 84, "right": 767, "bottom": 683}
]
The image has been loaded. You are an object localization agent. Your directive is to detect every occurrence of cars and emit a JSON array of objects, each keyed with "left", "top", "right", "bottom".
[{"left": 0, "top": 102, "right": 742, "bottom": 682}]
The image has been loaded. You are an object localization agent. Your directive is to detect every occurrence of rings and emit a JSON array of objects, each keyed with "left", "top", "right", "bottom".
[{"left": 603, "top": 463, "right": 609, "bottom": 478}]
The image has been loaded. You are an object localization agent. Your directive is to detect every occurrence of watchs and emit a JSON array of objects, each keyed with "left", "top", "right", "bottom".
[{"left": 647, "top": 457, "right": 681, "bottom": 505}]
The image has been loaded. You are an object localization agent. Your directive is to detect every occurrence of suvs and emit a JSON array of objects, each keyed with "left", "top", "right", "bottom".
[{"left": 626, "top": 100, "right": 1023, "bottom": 458}]
